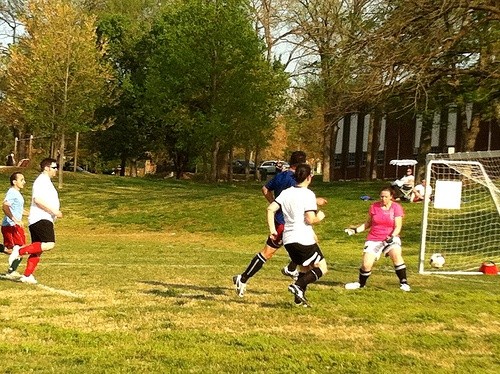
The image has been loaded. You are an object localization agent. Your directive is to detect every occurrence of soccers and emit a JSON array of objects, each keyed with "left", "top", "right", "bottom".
[{"left": 430, "top": 253, "right": 445, "bottom": 268}]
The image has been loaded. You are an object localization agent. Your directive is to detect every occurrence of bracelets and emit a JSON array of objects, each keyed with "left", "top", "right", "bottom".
[{"left": 355, "top": 228, "right": 356, "bottom": 233}]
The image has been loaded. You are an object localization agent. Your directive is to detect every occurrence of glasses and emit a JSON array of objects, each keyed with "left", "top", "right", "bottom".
[{"left": 52, "top": 166, "right": 57, "bottom": 169}]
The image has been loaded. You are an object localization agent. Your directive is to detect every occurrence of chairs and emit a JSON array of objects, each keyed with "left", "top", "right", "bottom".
[{"left": 390, "top": 159, "right": 418, "bottom": 201}]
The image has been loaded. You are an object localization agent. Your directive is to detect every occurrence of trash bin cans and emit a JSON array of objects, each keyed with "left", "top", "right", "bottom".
[{"left": 260, "top": 168, "right": 267, "bottom": 182}]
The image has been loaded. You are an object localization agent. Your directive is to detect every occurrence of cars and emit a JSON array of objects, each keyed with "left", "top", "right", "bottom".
[{"left": 233, "top": 160, "right": 289, "bottom": 175}]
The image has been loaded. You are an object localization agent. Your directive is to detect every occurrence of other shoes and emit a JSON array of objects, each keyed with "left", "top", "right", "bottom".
[
  {"left": 20, "top": 274, "right": 36, "bottom": 284},
  {"left": 232, "top": 275, "right": 246, "bottom": 298},
  {"left": 288, "top": 284, "right": 307, "bottom": 305},
  {"left": 5, "top": 270, "right": 20, "bottom": 277},
  {"left": 345, "top": 282, "right": 360, "bottom": 290},
  {"left": 9, "top": 244, "right": 20, "bottom": 266},
  {"left": 399, "top": 283, "right": 412, "bottom": 291},
  {"left": 281, "top": 266, "right": 299, "bottom": 280}
]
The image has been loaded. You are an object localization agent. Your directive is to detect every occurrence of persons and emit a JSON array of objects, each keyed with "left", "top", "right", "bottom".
[
  {"left": 405, "top": 179, "right": 432, "bottom": 203},
  {"left": 276, "top": 159, "right": 283, "bottom": 174},
  {"left": 6, "top": 152, "right": 15, "bottom": 166},
  {"left": 400, "top": 169, "right": 414, "bottom": 189},
  {"left": 345, "top": 186, "right": 411, "bottom": 292},
  {"left": 9, "top": 158, "right": 63, "bottom": 284},
  {"left": 0, "top": 172, "right": 26, "bottom": 278},
  {"left": 266, "top": 164, "right": 328, "bottom": 307},
  {"left": 233, "top": 151, "right": 328, "bottom": 298}
]
